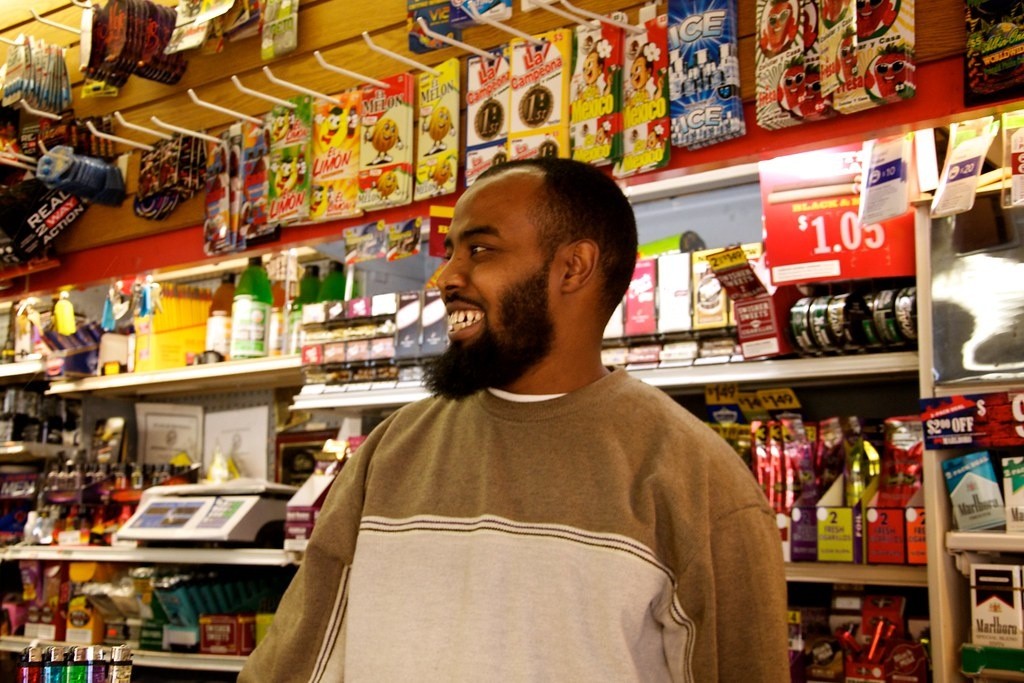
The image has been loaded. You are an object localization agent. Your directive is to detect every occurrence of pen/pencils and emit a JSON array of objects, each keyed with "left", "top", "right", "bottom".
[{"left": 867, "top": 619, "right": 884, "bottom": 661}]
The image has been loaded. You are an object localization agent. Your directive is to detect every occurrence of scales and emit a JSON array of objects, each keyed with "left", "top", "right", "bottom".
[{"left": 116, "top": 482, "right": 301, "bottom": 542}]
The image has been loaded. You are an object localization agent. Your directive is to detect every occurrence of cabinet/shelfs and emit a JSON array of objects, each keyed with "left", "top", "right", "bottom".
[{"left": 0, "top": 0, "right": 1024, "bottom": 683}]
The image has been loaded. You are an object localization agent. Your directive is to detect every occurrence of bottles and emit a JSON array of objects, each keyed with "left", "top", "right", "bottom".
[
  {"left": 229, "top": 256, "right": 274, "bottom": 360},
  {"left": 288, "top": 265, "right": 318, "bottom": 351},
  {"left": 316, "top": 260, "right": 359, "bottom": 302},
  {"left": 203, "top": 271, "right": 234, "bottom": 366}
]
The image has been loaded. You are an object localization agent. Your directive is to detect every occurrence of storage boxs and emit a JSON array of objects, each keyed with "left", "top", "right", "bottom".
[
  {"left": 65, "top": 605, "right": 105, "bottom": 646},
  {"left": 701, "top": 244, "right": 801, "bottom": 359},
  {"left": 969, "top": 564, "right": 1022, "bottom": 650},
  {"left": 200, "top": 617, "right": 256, "bottom": 654}
]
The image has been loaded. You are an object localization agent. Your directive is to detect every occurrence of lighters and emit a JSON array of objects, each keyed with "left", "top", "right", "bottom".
[{"left": 19, "top": 646, "right": 134, "bottom": 683}]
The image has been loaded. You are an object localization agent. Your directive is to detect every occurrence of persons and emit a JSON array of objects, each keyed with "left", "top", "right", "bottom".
[{"left": 236, "top": 157, "right": 790, "bottom": 683}]
